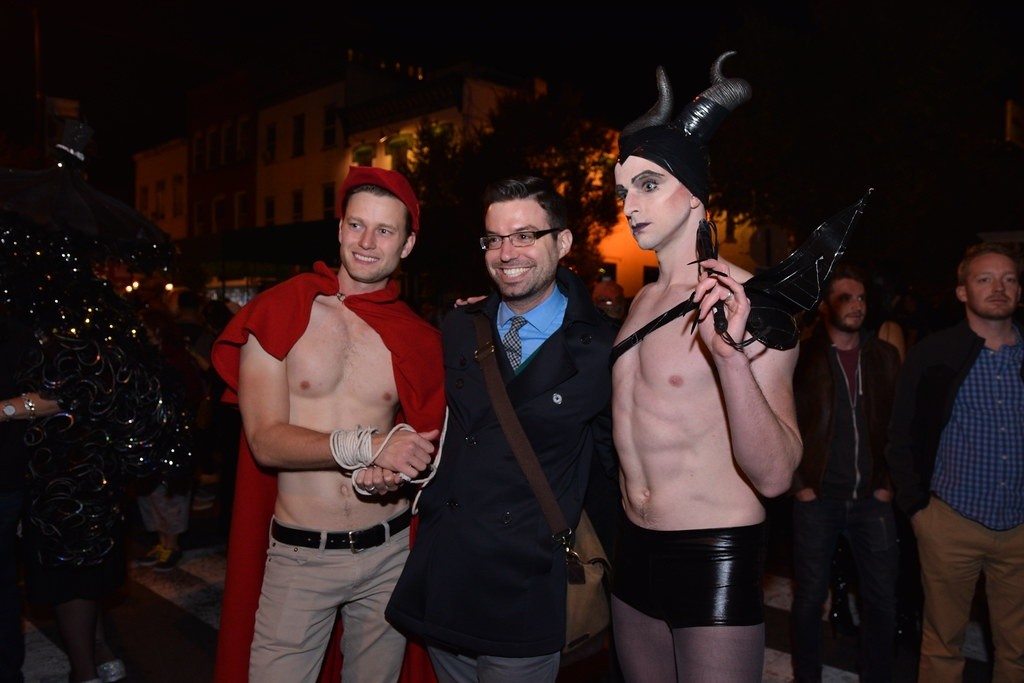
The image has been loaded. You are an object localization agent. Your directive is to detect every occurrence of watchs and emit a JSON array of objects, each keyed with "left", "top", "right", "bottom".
[{"left": 2, "top": 400, "right": 16, "bottom": 422}]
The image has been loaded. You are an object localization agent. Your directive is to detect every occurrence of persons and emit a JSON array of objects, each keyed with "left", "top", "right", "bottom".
[
  {"left": 454, "top": 123, "right": 803, "bottom": 683},
  {"left": 884, "top": 244, "right": 1024, "bottom": 683},
  {"left": 212, "top": 166, "right": 446, "bottom": 683},
  {"left": 383, "top": 174, "right": 620, "bottom": 683},
  {"left": 0, "top": 208, "right": 201, "bottom": 683},
  {"left": 791, "top": 270, "right": 904, "bottom": 683}
]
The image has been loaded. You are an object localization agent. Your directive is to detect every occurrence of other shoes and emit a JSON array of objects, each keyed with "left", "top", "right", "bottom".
[
  {"left": 83, "top": 659, "right": 126, "bottom": 683},
  {"left": 151, "top": 543, "right": 181, "bottom": 571},
  {"left": 136, "top": 544, "right": 164, "bottom": 567}
]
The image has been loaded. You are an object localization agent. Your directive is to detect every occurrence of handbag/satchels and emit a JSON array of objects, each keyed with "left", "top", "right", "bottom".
[{"left": 562, "top": 509, "right": 611, "bottom": 653}]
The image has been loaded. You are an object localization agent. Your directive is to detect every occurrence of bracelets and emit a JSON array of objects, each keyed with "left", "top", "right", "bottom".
[{"left": 21, "top": 392, "right": 35, "bottom": 422}]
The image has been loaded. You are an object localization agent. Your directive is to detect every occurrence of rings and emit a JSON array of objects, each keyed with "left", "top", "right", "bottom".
[
  {"left": 365, "top": 487, "right": 375, "bottom": 491},
  {"left": 724, "top": 290, "right": 732, "bottom": 302}
]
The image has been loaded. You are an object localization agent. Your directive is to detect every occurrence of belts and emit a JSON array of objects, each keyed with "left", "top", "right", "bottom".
[{"left": 272, "top": 507, "right": 413, "bottom": 554}]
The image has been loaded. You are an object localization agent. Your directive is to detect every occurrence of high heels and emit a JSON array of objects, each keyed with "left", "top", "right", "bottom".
[
  {"left": 829, "top": 594, "right": 859, "bottom": 639},
  {"left": 892, "top": 601, "right": 920, "bottom": 656}
]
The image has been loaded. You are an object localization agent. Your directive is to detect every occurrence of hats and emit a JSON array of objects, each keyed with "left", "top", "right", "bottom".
[{"left": 337, "top": 165, "right": 420, "bottom": 235}]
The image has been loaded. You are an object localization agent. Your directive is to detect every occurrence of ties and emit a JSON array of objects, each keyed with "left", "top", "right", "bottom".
[{"left": 503, "top": 316, "right": 526, "bottom": 370}]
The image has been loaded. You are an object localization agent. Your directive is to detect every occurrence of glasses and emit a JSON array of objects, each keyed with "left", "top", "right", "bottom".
[{"left": 479, "top": 227, "right": 565, "bottom": 250}]
